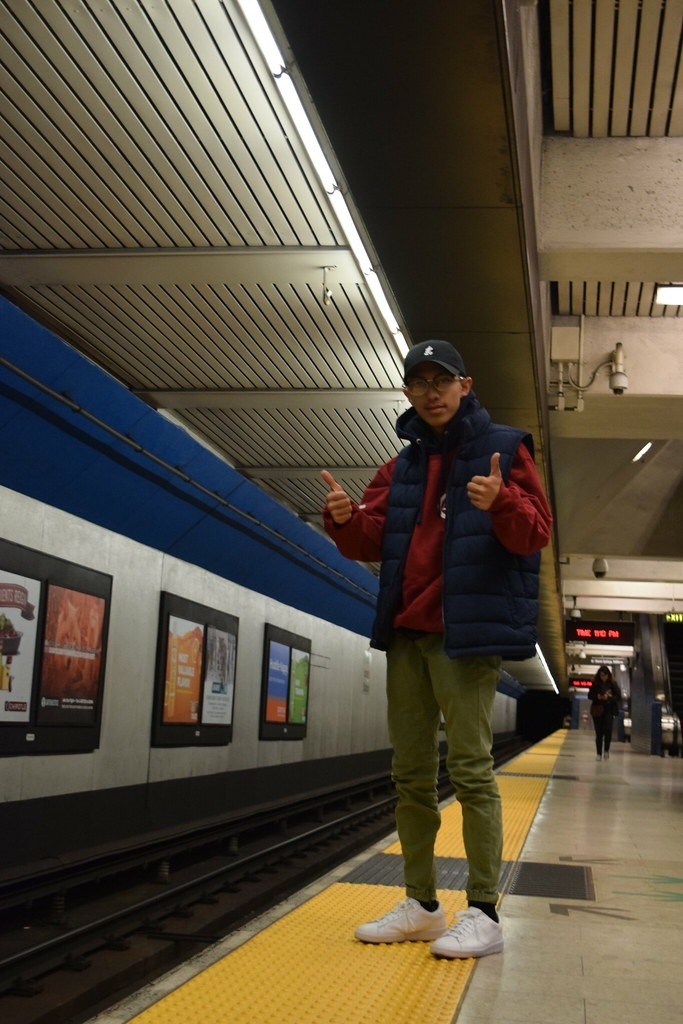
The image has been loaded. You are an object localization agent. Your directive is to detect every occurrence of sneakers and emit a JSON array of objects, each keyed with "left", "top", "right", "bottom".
[
  {"left": 430, "top": 907, "right": 504, "bottom": 958},
  {"left": 354, "top": 896, "right": 448, "bottom": 943}
]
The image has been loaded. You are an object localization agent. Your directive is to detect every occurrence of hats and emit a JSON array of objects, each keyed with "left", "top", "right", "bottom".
[{"left": 404, "top": 340, "right": 466, "bottom": 387}]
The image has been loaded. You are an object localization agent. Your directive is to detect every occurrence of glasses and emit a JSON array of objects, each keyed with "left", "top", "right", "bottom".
[{"left": 407, "top": 373, "right": 465, "bottom": 396}]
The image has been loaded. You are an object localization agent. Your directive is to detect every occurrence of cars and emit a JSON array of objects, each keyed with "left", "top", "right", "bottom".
[{"left": 618, "top": 697, "right": 680, "bottom": 739}]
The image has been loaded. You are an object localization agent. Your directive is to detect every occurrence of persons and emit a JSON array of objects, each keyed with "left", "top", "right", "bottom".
[
  {"left": 587, "top": 667, "right": 622, "bottom": 760},
  {"left": 321, "top": 341, "right": 552, "bottom": 958}
]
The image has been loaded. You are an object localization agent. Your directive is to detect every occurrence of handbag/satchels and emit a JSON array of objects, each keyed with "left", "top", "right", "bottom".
[{"left": 612, "top": 681, "right": 619, "bottom": 717}]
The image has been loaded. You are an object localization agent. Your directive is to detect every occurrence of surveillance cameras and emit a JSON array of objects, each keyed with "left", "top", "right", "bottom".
[
  {"left": 592, "top": 559, "right": 608, "bottom": 578},
  {"left": 571, "top": 610, "right": 581, "bottom": 622},
  {"left": 609, "top": 372, "right": 629, "bottom": 394}
]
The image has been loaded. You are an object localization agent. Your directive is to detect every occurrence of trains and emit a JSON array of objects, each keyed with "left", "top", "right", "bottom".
[{"left": 0, "top": 292, "right": 524, "bottom": 876}]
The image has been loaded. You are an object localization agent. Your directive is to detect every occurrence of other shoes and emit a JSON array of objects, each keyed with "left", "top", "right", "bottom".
[
  {"left": 596, "top": 752, "right": 602, "bottom": 761},
  {"left": 605, "top": 750, "right": 609, "bottom": 760}
]
